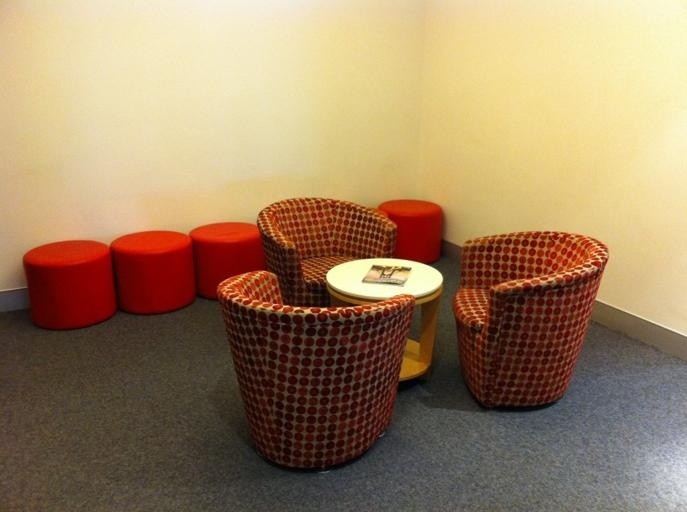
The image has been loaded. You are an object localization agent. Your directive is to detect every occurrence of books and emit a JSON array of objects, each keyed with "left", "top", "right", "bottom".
[{"left": 362, "top": 265, "right": 412, "bottom": 285}]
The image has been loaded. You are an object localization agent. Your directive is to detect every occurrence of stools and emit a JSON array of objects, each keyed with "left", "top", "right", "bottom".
[
  {"left": 112, "top": 231, "right": 198, "bottom": 316},
  {"left": 190, "top": 222, "right": 266, "bottom": 300},
  {"left": 22, "top": 241, "right": 119, "bottom": 330},
  {"left": 379, "top": 200, "right": 443, "bottom": 265}
]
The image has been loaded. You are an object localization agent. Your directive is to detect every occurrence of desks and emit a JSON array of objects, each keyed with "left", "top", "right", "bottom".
[{"left": 326, "top": 258, "right": 443, "bottom": 382}]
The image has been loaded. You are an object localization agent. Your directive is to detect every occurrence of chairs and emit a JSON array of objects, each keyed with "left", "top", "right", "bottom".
[
  {"left": 217, "top": 270, "right": 415, "bottom": 471},
  {"left": 258, "top": 198, "right": 397, "bottom": 307},
  {"left": 452, "top": 231, "right": 609, "bottom": 409}
]
